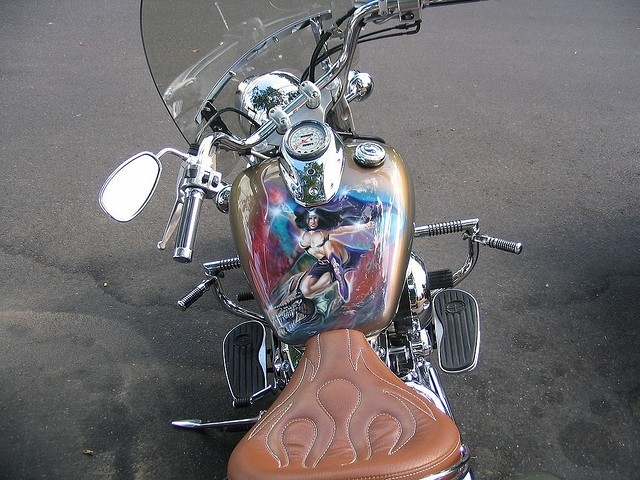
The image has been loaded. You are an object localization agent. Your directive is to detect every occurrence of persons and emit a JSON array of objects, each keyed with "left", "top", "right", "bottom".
[{"left": 283, "top": 208, "right": 376, "bottom": 303}]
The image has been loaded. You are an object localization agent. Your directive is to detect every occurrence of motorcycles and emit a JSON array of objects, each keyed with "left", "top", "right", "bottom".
[{"left": 98, "top": 0, "right": 523, "bottom": 480}]
